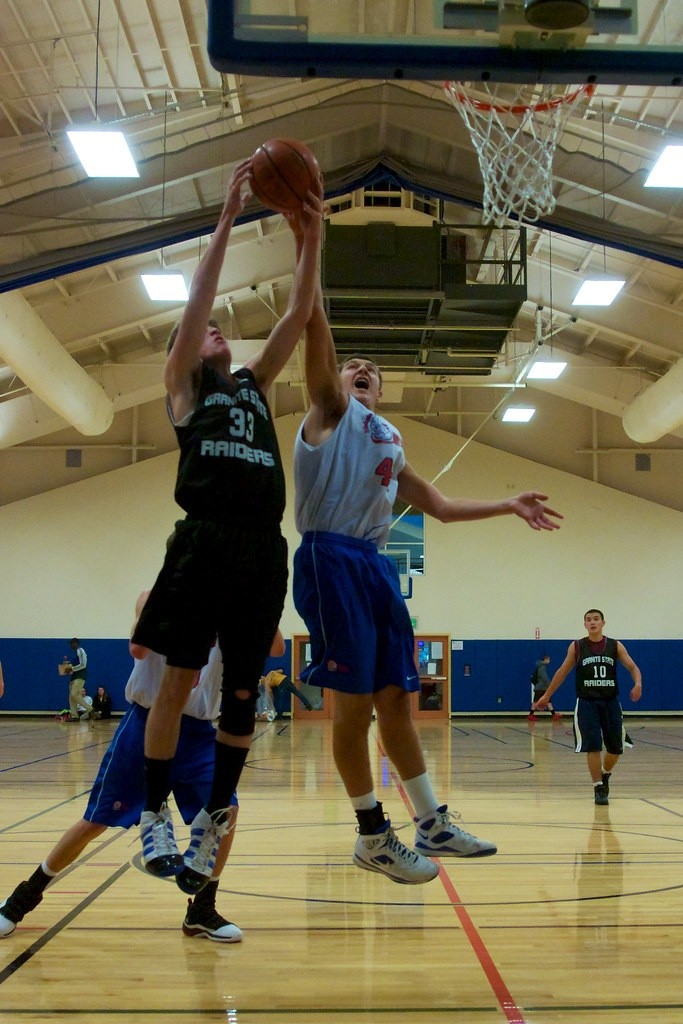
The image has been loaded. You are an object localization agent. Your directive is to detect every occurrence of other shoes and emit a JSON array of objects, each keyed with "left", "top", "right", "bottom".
[
  {"left": 602, "top": 772, "right": 611, "bottom": 786},
  {"left": 553, "top": 714, "right": 560, "bottom": 720},
  {"left": 66, "top": 718, "right": 79, "bottom": 722},
  {"left": 594, "top": 785, "right": 608, "bottom": 804},
  {"left": 528, "top": 715, "right": 539, "bottom": 721}
]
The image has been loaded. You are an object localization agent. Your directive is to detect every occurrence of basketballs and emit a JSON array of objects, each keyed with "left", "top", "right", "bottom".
[{"left": 248, "top": 137, "right": 320, "bottom": 213}]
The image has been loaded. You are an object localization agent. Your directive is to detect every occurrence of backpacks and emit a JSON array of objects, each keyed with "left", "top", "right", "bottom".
[{"left": 531, "top": 667, "right": 538, "bottom": 685}]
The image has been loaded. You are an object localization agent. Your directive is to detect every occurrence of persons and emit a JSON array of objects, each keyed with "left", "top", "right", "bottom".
[
  {"left": 89, "top": 686, "right": 112, "bottom": 720},
  {"left": 64, "top": 638, "right": 95, "bottom": 722},
  {"left": 59, "top": 688, "right": 93, "bottom": 720},
  {"left": 0, "top": 523, "right": 286, "bottom": 944},
  {"left": 131, "top": 157, "right": 324, "bottom": 895},
  {"left": 282, "top": 210, "right": 564, "bottom": 886},
  {"left": 265, "top": 669, "right": 312, "bottom": 721},
  {"left": 536, "top": 609, "right": 642, "bottom": 804},
  {"left": 528, "top": 656, "right": 562, "bottom": 721}
]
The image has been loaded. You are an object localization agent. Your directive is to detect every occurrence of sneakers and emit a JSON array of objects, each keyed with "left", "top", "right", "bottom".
[
  {"left": 127, "top": 802, "right": 183, "bottom": 877},
  {"left": 413, "top": 804, "right": 497, "bottom": 857},
  {"left": 182, "top": 898, "right": 243, "bottom": 942},
  {"left": 0, "top": 881, "right": 42, "bottom": 937},
  {"left": 176, "top": 805, "right": 236, "bottom": 894},
  {"left": 352, "top": 812, "right": 439, "bottom": 885}
]
